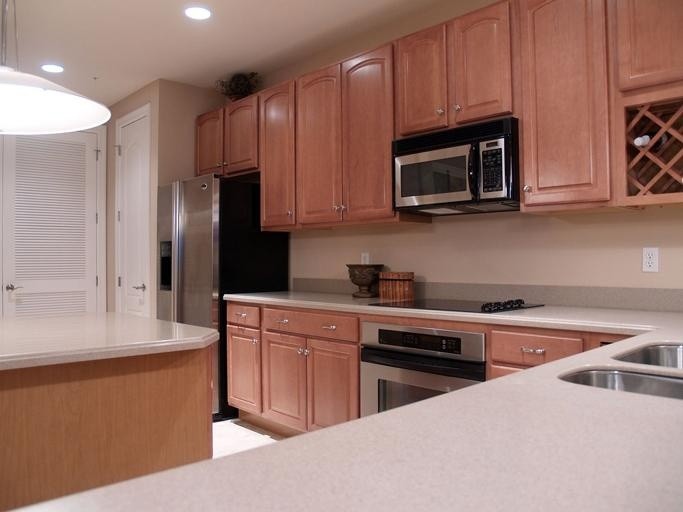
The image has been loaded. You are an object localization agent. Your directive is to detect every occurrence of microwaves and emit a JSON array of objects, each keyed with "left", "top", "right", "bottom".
[{"left": 392, "top": 117, "right": 521, "bottom": 217}]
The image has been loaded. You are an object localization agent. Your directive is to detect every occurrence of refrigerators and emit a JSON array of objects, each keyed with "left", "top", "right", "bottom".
[{"left": 172, "top": 173, "right": 289, "bottom": 422}]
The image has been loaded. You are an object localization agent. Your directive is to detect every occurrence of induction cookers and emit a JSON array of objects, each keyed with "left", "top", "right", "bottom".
[{"left": 368, "top": 298, "right": 546, "bottom": 314}]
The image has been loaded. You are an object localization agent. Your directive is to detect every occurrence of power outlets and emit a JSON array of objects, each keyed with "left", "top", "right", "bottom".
[{"left": 640, "top": 246, "right": 660, "bottom": 273}]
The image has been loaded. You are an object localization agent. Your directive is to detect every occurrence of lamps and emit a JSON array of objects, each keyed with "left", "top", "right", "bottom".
[{"left": 0, "top": 0, "right": 112, "bottom": 137}]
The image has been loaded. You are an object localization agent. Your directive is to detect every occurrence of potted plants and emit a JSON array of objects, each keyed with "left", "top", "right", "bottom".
[{"left": 215, "top": 72, "right": 264, "bottom": 102}]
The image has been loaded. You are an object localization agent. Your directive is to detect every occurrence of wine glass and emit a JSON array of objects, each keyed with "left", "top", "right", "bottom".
[{"left": 346, "top": 264, "right": 384, "bottom": 298}]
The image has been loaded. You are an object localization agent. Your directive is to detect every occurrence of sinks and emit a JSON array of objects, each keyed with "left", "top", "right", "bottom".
[
  {"left": 609, "top": 339, "right": 683, "bottom": 372},
  {"left": 556, "top": 364, "right": 683, "bottom": 402}
]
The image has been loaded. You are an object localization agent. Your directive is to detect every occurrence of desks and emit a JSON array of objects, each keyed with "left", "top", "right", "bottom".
[{"left": 0, "top": 311, "right": 222, "bottom": 512}]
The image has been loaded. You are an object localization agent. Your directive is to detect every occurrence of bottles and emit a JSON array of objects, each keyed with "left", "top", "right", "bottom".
[{"left": 635, "top": 132, "right": 672, "bottom": 148}]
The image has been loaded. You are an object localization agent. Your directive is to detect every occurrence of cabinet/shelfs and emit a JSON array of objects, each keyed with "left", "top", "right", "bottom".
[
  {"left": 259, "top": 300, "right": 360, "bottom": 438},
  {"left": 506, "top": 1, "right": 612, "bottom": 215},
  {"left": 604, "top": 0, "right": 682, "bottom": 209},
  {"left": 257, "top": 76, "right": 298, "bottom": 233},
  {"left": 294, "top": 30, "right": 433, "bottom": 232},
  {"left": 223, "top": 298, "right": 262, "bottom": 428},
  {"left": 194, "top": 93, "right": 260, "bottom": 179},
  {"left": 390, "top": 1, "right": 516, "bottom": 141},
  {"left": 485, "top": 324, "right": 590, "bottom": 382}
]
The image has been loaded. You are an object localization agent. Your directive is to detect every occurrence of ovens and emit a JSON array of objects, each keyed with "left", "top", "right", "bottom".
[{"left": 361, "top": 321, "right": 486, "bottom": 417}]
